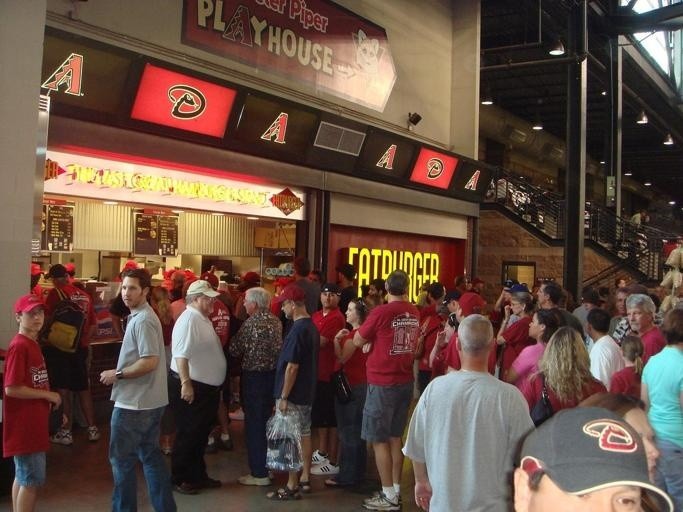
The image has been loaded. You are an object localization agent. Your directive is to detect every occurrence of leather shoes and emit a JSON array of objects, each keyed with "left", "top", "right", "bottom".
[{"left": 198, "top": 477, "right": 224, "bottom": 490}]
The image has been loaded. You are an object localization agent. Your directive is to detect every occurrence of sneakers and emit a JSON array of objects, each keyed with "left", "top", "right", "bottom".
[
  {"left": 239, "top": 474, "right": 271, "bottom": 488},
  {"left": 367, "top": 487, "right": 386, "bottom": 498},
  {"left": 88, "top": 424, "right": 101, "bottom": 444},
  {"left": 362, "top": 494, "right": 404, "bottom": 512},
  {"left": 228, "top": 408, "right": 247, "bottom": 422},
  {"left": 308, "top": 458, "right": 342, "bottom": 476},
  {"left": 325, "top": 476, "right": 359, "bottom": 485},
  {"left": 307, "top": 450, "right": 330, "bottom": 464},
  {"left": 49, "top": 429, "right": 76, "bottom": 448},
  {"left": 218, "top": 433, "right": 234, "bottom": 450}
]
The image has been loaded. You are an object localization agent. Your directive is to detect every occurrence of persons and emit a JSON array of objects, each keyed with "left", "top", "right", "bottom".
[
  {"left": 496, "top": 290, "right": 534, "bottom": 382},
  {"left": 169, "top": 280, "right": 227, "bottom": 495},
  {"left": 149, "top": 286, "right": 176, "bottom": 455},
  {"left": 400, "top": 313, "right": 535, "bottom": 511},
  {"left": 582, "top": 291, "right": 601, "bottom": 311},
  {"left": 30, "top": 263, "right": 48, "bottom": 304},
  {"left": 599, "top": 285, "right": 611, "bottom": 300},
  {"left": 369, "top": 278, "right": 387, "bottom": 306},
  {"left": 3, "top": 293, "right": 61, "bottom": 510},
  {"left": 414, "top": 302, "right": 448, "bottom": 397},
  {"left": 223, "top": 287, "right": 281, "bottom": 486},
  {"left": 100, "top": 270, "right": 176, "bottom": 511},
  {"left": 430, "top": 293, "right": 497, "bottom": 377},
  {"left": 418, "top": 282, "right": 446, "bottom": 323},
  {"left": 611, "top": 335, "right": 643, "bottom": 407},
  {"left": 534, "top": 282, "right": 567, "bottom": 327},
  {"left": 266, "top": 285, "right": 320, "bottom": 501},
  {"left": 326, "top": 296, "right": 368, "bottom": 489},
  {"left": 200, "top": 272, "right": 231, "bottom": 454},
  {"left": 416, "top": 276, "right": 486, "bottom": 300},
  {"left": 115, "top": 260, "right": 139, "bottom": 282},
  {"left": 334, "top": 263, "right": 356, "bottom": 329},
  {"left": 522, "top": 327, "right": 608, "bottom": 427},
  {"left": 43, "top": 263, "right": 99, "bottom": 444},
  {"left": 571, "top": 286, "right": 595, "bottom": 335},
  {"left": 576, "top": 392, "right": 681, "bottom": 511},
  {"left": 586, "top": 309, "right": 625, "bottom": 391},
  {"left": 661, "top": 235, "right": 677, "bottom": 274},
  {"left": 493, "top": 282, "right": 531, "bottom": 322},
  {"left": 289, "top": 258, "right": 320, "bottom": 315},
  {"left": 614, "top": 278, "right": 627, "bottom": 290},
  {"left": 557, "top": 289, "right": 585, "bottom": 341},
  {"left": 65, "top": 263, "right": 86, "bottom": 290},
  {"left": 640, "top": 310, "right": 682, "bottom": 511},
  {"left": 626, "top": 294, "right": 667, "bottom": 364},
  {"left": 353, "top": 270, "right": 419, "bottom": 512},
  {"left": 311, "top": 284, "right": 346, "bottom": 476},
  {"left": 161, "top": 269, "right": 198, "bottom": 303},
  {"left": 230, "top": 272, "right": 261, "bottom": 419},
  {"left": 502, "top": 309, "right": 560, "bottom": 426},
  {"left": 632, "top": 209, "right": 647, "bottom": 252}
]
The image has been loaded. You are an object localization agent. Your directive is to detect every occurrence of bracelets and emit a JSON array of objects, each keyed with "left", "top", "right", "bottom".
[
  {"left": 116, "top": 369, "right": 123, "bottom": 379},
  {"left": 179, "top": 375, "right": 191, "bottom": 386}
]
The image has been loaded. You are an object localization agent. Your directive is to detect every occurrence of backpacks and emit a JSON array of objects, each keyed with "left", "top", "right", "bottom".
[{"left": 531, "top": 372, "right": 554, "bottom": 426}]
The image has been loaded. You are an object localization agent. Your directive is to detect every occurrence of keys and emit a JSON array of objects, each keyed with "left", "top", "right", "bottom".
[{"left": 614, "top": 287, "right": 638, "bottom": 317}]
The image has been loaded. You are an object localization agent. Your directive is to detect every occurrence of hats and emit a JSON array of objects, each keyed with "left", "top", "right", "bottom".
[
  {"left": 240, "top": 272, "right": 261, "bottom": 284},
  {"left": 273, "top": 278, "right": 295, "bottom": 285},
  {"left": 443, "top": 290, "right": 461, "bottom": 303},
  {"left": 118, "top": 262, "right": 139, "bottom": 277},
  {"left": 14, "top": 294, "right": 50, "bottom": 314},
  {"left": 30, "top": 263, "right": 40, "bottom": 278},
  {"left": 504, "top": 283, "right": 529, "bottom": 293},
  {"left": 44, "top": 264, "right": 70, "bottom": 281},
  {"left": 454, "top": 289, "right": 487, "bottom": 319},
  {"left": 185, "top": 277, "right": 221, "bottom": 299},
  {"left": 320, "top": 282, "right": 345, "bottom": 295},
  {"left": 272, "top": 284, "right": 306, "bottom": 302},
  {"left": 336, "top": 263, "right": 356, "bottom": 278}
]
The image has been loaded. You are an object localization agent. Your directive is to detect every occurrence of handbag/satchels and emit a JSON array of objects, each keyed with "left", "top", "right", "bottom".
[{"left": 332, "top": 361, "right": 354, "bottom": 405}]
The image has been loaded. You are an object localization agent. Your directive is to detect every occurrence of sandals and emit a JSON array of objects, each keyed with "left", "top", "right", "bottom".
[
  {"left": 299, "top": 480, "right": 312, "bottom": 495},
  {"left": 265, "top": 484, "right": 302, "bottom": 500},
  {"left": 169, "top": 478, "right": 200, "bottom": 498}
]
